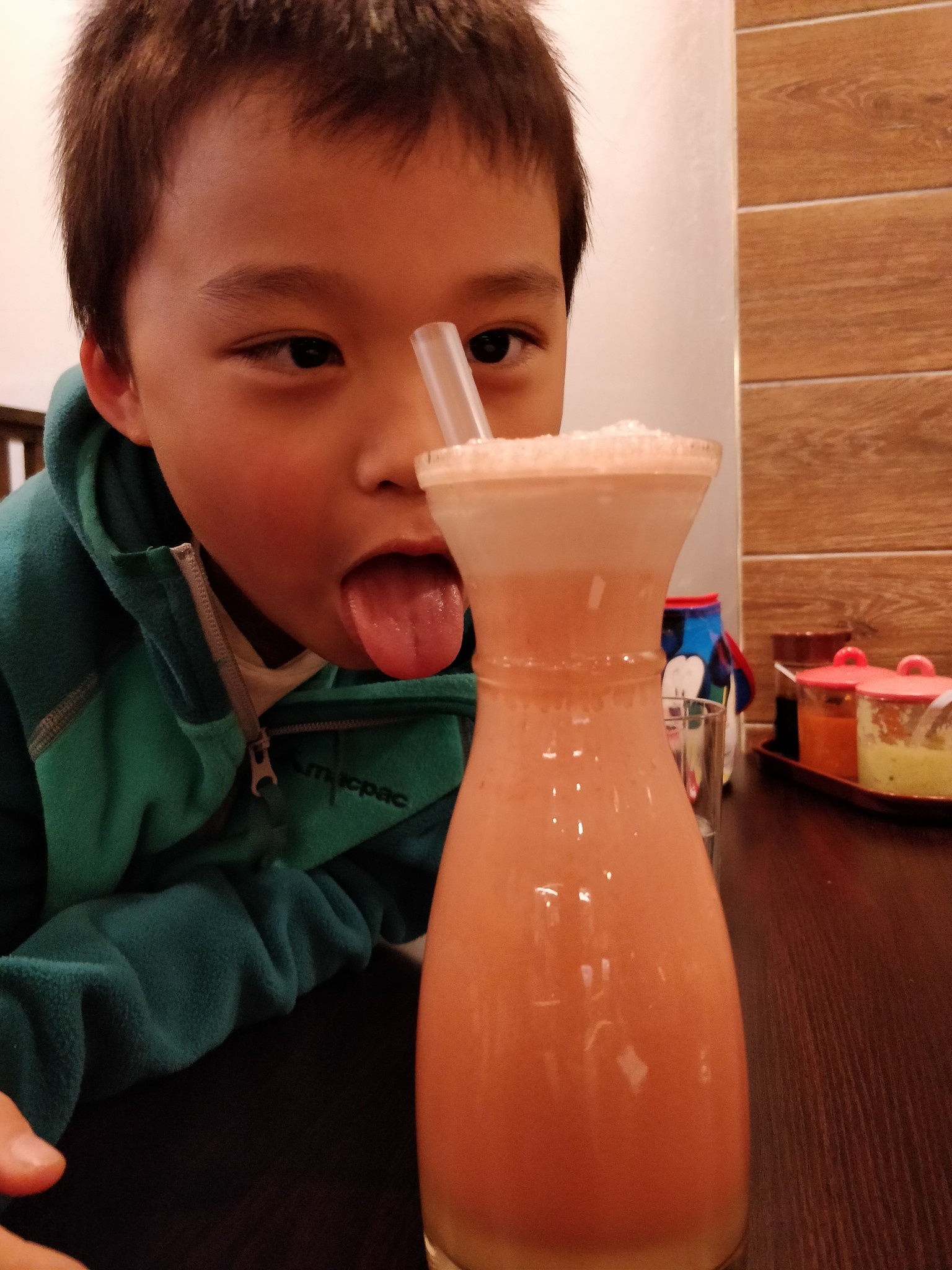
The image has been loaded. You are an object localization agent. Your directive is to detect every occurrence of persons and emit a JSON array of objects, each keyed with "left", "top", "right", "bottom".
[{"left": 0, "top": 0, "right": 588, "bottom": 1270}]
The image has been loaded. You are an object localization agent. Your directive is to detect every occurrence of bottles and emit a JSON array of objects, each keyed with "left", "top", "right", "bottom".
[
  {"left": 771, "top": 628, "right": 852, "bottom": 762},
  {"left": 414, "top": 434, "right": 751, "bottom": 1270}
]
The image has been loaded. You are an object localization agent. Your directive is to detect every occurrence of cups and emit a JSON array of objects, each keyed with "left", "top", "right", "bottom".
[
  {"left": 796, "top": 646, "right": 897, "bottom": 784},
  {"left": 855, "top": 655, "right": 952, "bottom": 800},
  {"left": 662, "top": 696, "right": 727, "bottom": 890}
]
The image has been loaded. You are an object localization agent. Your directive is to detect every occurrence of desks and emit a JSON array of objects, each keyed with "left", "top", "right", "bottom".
[{"left": 22, "top": 739, "right": 952, "bottom": 1270}]
